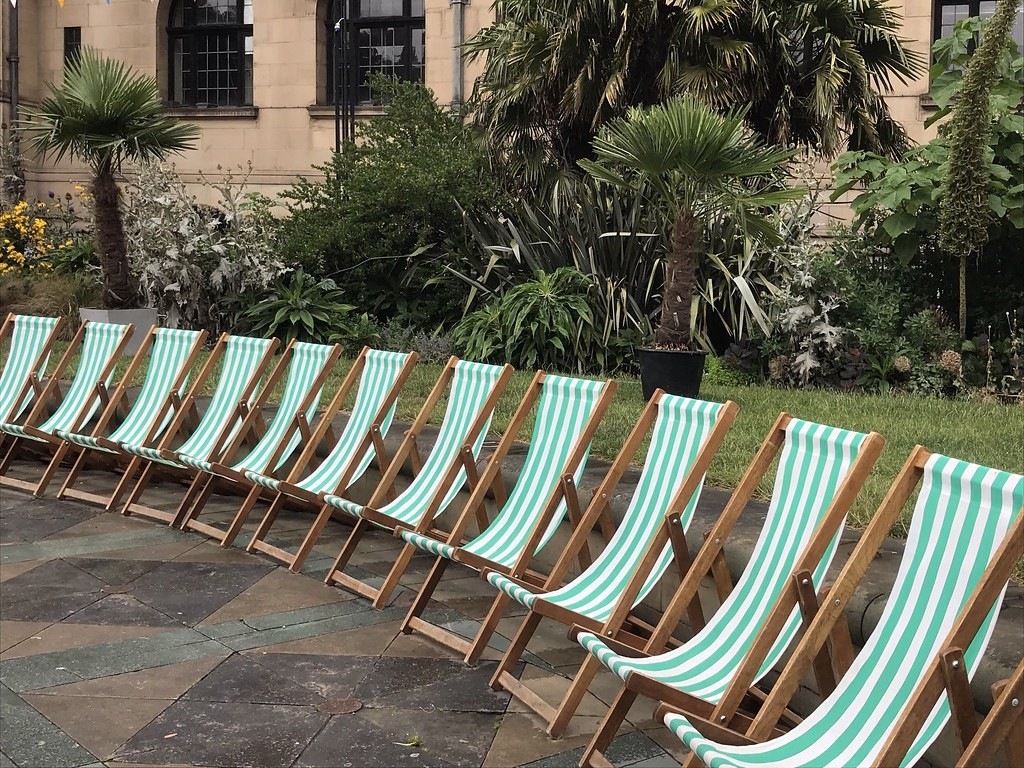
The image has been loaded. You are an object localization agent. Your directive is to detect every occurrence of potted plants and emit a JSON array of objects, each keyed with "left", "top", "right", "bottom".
[
  {"left": 7, "top": 43, "right": 201, "bottom": 357},
  {"left": 572, "top": 90, "right": 810, "bottom": 401}
]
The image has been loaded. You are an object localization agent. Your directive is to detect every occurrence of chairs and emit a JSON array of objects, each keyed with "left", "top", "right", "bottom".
[{"left": 0, "top": 311, "right": 1024, "bottom": 768}]
